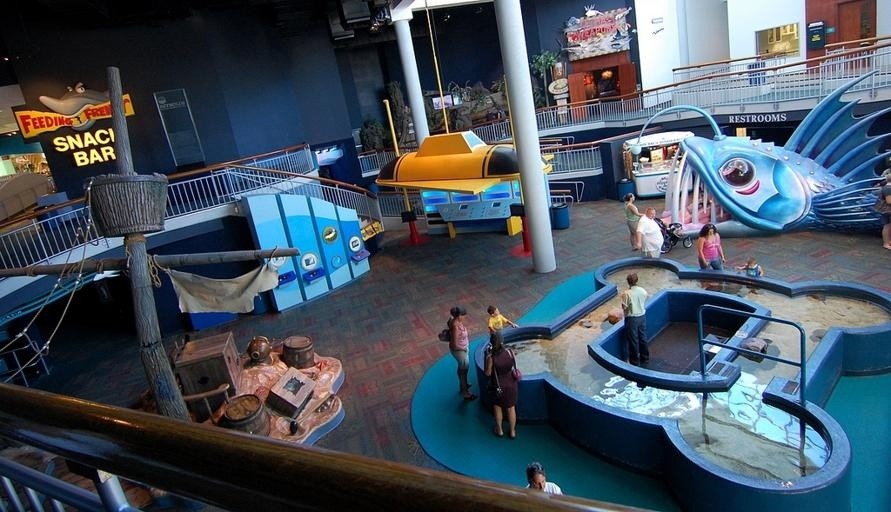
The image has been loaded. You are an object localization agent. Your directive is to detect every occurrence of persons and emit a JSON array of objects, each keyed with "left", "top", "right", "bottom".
[
  {"left": 881, "top": 173, "right": 891, "bottom": 250},
  {"left": 486, "top": 304, "right": 519, "bottom": 336},
  {"left": 735, "top": 257, "right": 763, "bottom": 278},
  {"left": 634, "top": 208, "right": 664, "bottom": 259},
  {"left": 524, "top": 461, "right": 564, "bottom": 496},
  {"left": 697, "top": 224, "right": 726, "bottom": 272},
  {"left": 621, "top": 271, "right": 650, "bottom": 367},
  {"left": 483, "top": 331, "right": 518, "bottom": 439},
  {"left": 448, "top": 306, "right": 478, "bottom": 401},
  {"left": 622, "top": 192, "right": 645, "bottom": 251}
]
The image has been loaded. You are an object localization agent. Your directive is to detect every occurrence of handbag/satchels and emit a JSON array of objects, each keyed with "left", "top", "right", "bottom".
[
  {"left": 438, "top": 329, "right": 451, "bottom": 342},
  {"left": 511, "top": 368, "right": 522, "bottom": 383},
  {"left": 480, "top": 384, "right": 504, "bottom": 401}
]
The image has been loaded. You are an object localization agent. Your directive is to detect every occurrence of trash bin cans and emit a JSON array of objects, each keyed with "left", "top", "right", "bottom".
[
  {"left": 616, "top": 179, "right": 635, "bottom": 202},
  {"left": 367, "top": 182, "right": 379, "bottom": 193},
  {"left": 32, "top": 204, "right": 61, "bottom": 230},
  {"left": 747, "top": 61, "right": 765, "bottom": 84}
]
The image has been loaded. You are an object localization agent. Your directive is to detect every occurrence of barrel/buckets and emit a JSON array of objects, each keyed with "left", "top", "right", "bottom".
[
  {"left": 283, "top": 335, "right": 314, "bottom": 370},
  {"left": 618, "top": 177, "right": 635, "bottom": 201},
  {"left": 225, "top": 394, "right": 269, "bottom": 437}
]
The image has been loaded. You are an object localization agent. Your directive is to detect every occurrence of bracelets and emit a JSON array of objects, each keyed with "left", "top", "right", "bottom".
[{"left": 721, "top": 256, "right": 724, "bottom": 258}]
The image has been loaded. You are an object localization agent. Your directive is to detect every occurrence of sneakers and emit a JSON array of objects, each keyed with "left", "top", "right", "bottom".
[{"left": 460, "top": 383, "right": 478, "bottom": 401}]
